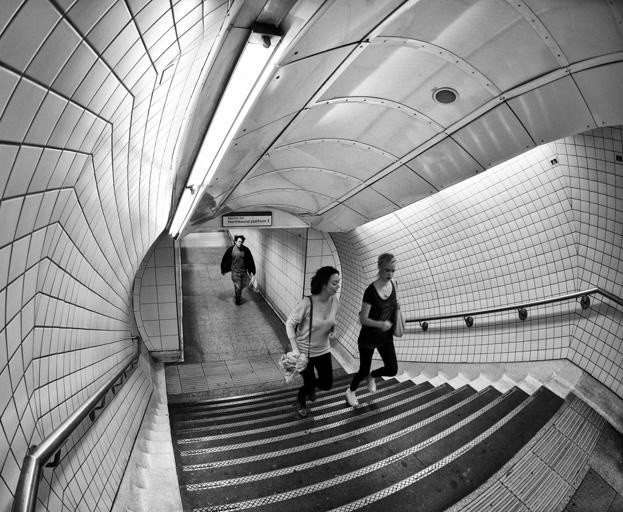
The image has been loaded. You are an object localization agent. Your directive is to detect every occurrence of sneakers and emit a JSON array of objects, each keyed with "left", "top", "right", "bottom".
[
  {"left": 345, "top": 388, "right": 359, "bottom": 407},
  {"left": 366, "top": 370, "right": 376, "bottom": 393}
]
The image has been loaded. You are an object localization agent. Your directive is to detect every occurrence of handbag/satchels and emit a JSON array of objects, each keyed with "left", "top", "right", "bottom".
[
  {"left": 279, "top": 351, "right": 308, "bottom": 380},
  {"left": 394, "top": 309, "right": 404, "bottom": 337}
]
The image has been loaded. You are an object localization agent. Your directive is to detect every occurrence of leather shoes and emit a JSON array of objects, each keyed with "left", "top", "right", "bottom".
[{"left": 296, "top": 392, "right": 308, "bottom": 418}]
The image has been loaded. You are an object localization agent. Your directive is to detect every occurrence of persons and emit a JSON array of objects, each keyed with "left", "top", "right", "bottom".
[
  {"left": 221, "top": 234, "right": 256, "bottom": 305},
  {"left": 345, "top": 253, "right": 406, "bottom": 407},
  {"left": 285, "top": 265, "right": 341, "bottom": 417}
]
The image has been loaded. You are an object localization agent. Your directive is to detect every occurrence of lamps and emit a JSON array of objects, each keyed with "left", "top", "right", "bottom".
[{"left": 167, "top": 27, "right": 283, "bottom": 238}]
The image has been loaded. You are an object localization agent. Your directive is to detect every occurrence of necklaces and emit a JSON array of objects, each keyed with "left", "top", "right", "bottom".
[{"left": 382, "top": 286, "right": 388, "bottom": 297}]
